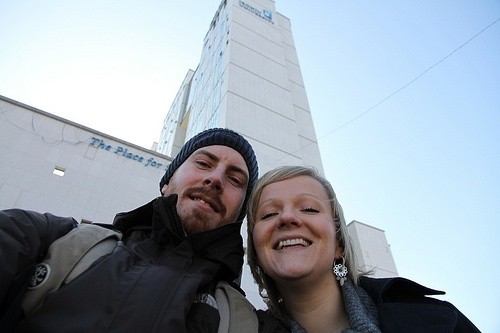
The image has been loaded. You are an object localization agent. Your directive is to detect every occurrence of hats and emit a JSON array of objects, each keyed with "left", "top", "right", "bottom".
[{"left": 160, "top": 128, "right": 257, "bottom": 222}]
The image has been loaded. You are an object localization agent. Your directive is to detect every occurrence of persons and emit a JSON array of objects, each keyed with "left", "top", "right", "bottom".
[
  {"left": 245, "top": 166, "right": 482, "bottom": 333},
  {"left": 0, "top": 128, "right": 286, "bottom": 333}
]
